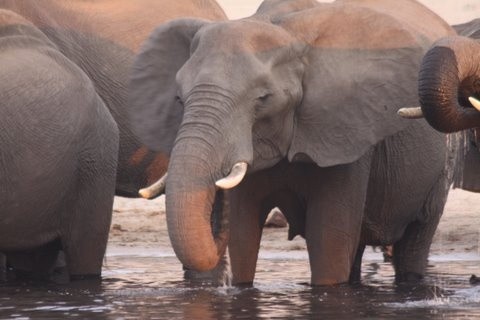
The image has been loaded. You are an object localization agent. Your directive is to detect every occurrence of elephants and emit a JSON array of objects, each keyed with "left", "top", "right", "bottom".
[{"left": 0, "top": 0, "right": 480, "bottom": 289}]
